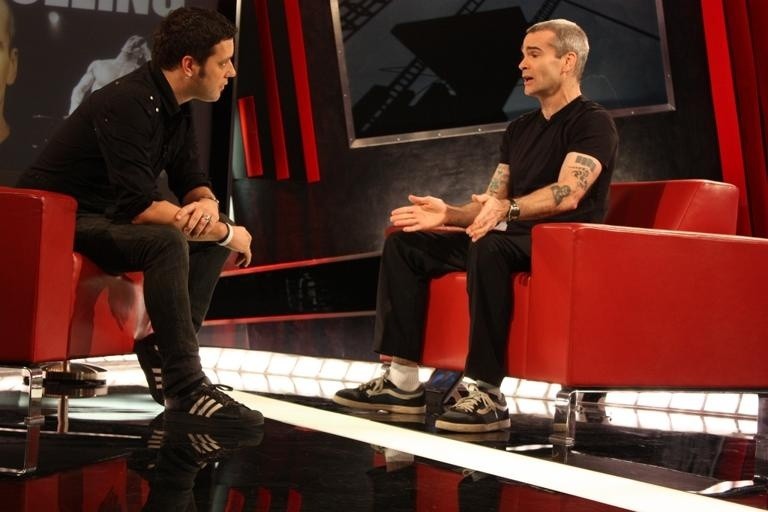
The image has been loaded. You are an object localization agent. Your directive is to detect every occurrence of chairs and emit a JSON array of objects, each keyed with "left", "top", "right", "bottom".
[
  {"left": 1, "top": 424, "right": 150, "bottom": 510},
  {"left": 0, "top": 186, "right": 151, "bottom": 420},
  {"left": 378, "top": 178, "right": 768, "bottom": 445}
]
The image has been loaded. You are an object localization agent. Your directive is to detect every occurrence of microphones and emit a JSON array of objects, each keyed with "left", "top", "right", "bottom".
[{"left": 132, "top": 39, "right": 145, "bottom": 47}]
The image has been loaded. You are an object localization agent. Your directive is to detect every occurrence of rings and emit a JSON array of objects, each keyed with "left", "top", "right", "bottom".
[{"left": 202, "top": 216, "right": 210, "bottom": 223}]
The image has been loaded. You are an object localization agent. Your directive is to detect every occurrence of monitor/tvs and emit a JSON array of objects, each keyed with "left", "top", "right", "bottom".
[{"left": 0, "top": 0, "right": 235, "bottom": 221}]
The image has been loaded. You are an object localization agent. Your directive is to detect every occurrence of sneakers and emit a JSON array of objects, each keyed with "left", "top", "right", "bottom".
[
  {"left": 162, "top": 384, "right": 265, "bottom": 426},
  {"left": 435, "top": 383, "right": 512, "bottom": 433},
  {"left": 331, "top": 376, "right": 428, "bottom": 415},
  {"left": 135, "top": 331, "right": 165, "bottom": 407}
]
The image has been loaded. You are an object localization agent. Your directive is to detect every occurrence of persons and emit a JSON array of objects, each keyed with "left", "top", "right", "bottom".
[
  {"left": 366, "top": 445, "right": 501, "bottom": 512},
  {"left": 0, "top": 1, "right": 32, "bottom": 171},
  {"left": 125, "top": 410, "right": 265, "bottom": 512},
  {"left": 332, "top": 19, "right": 619, "bottom": 434},
  {"left": 14, "top": 5, "right": 266, "bottom": 429},
  {"left": 68, "top": 34, "right": 152, "bottom": 115}
]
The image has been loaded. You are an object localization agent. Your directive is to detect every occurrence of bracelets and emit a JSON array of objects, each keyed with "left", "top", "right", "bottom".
[
  {"left": 216, "top": 222, "right": 234, "bottom": 247},
  {"left": 209, "top": 197, "right": 220, "bottom": 204}
]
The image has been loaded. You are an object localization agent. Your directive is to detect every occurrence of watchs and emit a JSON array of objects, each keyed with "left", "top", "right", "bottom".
[{"left": 508, "top": 199, "right": 520, "bottom": 223}]
